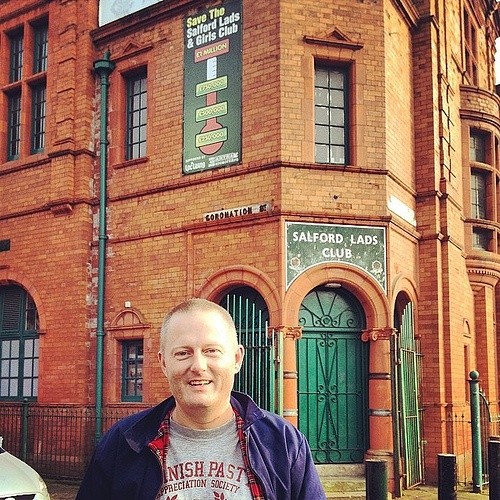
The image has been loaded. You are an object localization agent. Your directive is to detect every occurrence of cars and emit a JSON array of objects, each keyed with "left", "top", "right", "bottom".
[{"left": 0, "top": 437, "right": 50, "bottom": 500}]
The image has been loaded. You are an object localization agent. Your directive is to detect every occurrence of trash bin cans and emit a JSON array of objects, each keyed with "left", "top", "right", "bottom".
[{"left": 362, "top": 452, "right": 458, "bottom": 496}]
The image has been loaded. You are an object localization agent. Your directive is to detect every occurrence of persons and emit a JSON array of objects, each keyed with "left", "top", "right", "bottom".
[{"left": 76, "top": 298, "right": 327, "bottom": 499}]
